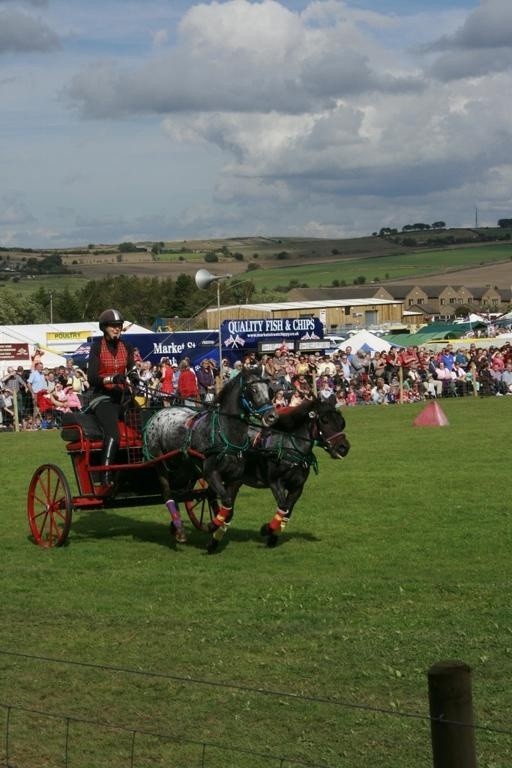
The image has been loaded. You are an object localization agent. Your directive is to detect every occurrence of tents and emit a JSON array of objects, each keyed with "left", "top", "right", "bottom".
[
  {"left": 1, "top": 318, "right": 157, "bottom": 366},
  {"left": 329, "top": 317, "right": 512, "bottom": 353}
]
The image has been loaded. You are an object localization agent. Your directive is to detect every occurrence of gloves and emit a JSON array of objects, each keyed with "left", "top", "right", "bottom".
[
  {"left": 113, "top": 374, "right": 126, "bottom": 384},
  {"left": 129, "top": 374, "right": 139, "bottom": 387}
]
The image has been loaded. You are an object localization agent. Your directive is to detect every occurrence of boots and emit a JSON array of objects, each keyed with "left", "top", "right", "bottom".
[{"left": 100, "top": 436, "right": 119, "bottom": 486}]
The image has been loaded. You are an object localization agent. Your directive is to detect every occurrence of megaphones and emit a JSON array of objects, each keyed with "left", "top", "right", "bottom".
[{"left": 194, "top": 267, "right": 231, "bottom": 292}]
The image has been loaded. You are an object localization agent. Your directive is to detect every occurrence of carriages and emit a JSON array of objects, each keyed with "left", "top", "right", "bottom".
[{"left": 28, "top": 361, "right": 350, "bottom": 553}]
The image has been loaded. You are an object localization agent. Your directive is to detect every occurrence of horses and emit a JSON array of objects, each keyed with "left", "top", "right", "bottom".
[
  {"left": 240, "top": 392, "right": 351, "bottom": 549},
  {"left": 144, "top": 359, "right": 280, "bottom": 555}
]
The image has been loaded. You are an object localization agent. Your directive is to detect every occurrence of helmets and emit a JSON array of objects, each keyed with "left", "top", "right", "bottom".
[{"left": 99, "top": 309, "right": 124, "bottom": 331}]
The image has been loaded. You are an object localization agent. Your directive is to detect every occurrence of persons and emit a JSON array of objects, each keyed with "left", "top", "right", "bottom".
[
  {"left": 287, "top": 343, "right": 511, "bottom": 407},
  {"left": 1, "top": 346, "right": 294, "bottom": 434},
  {"left": 87, "top": 308, "right": 149, "bottom": 499}
]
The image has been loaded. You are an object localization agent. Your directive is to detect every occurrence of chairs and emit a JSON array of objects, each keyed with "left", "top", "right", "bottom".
[{"left": 61, "top": 394, "right": 155, "bottom": 498}]
